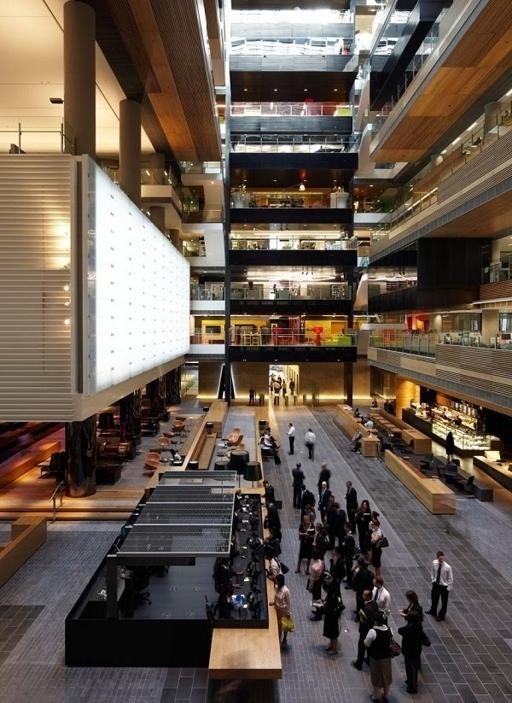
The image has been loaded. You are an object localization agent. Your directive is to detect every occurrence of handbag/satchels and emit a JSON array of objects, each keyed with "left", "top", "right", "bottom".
[
  {"left": 281, "top": 615, "right": 294, "bottom": 632},
  {"left": 334, "top": 604, "right": 345, "bottom": 613},
  {"left": 274, "top": 556, "right": 289, "bottom": 573},
  {"left": 376, "top": 536, "right": 389, "bottom": 548},
  {"left": 389, "top": 639, "right": 401, "bottom": 657},
  {"left": 422, "top": 630, "right": 431, "bottom": 645}
]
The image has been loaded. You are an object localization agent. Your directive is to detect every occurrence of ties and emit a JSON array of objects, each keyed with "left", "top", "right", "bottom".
[
  {"left": 375, "top": 588, "right": 378, "bottom": 600},
  {"left": 436, "top": 563, "right": 441, "bottom": 584}
]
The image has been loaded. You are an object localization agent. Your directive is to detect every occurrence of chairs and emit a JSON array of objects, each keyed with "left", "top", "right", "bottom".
[
  {"left": 145, "top": 416, "right": 243, "bottom": 477},
  {"left": 378, "top": 432, "right": 411, "bottom": 456},
  {"left": 420, "top": 452, "right": 475, "bottom": 493}
]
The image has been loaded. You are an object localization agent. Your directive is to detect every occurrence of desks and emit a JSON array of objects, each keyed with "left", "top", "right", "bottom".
[{"left": 369, "top": 411, "right": 401, "bottom": 432}]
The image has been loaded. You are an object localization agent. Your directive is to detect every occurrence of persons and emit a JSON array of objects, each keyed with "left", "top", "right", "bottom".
[
  {"left": 256, "top": 420, "right": 433, "bottom": 701},
  {"left": 273, "top": 284, "right": 280, "bottom": 300},
  {"left": 340, "top": 286, "right": 347, "bottom": 299},
  {"left": 260, "top": 326, "right": 265, "bottom": 345},
  {"left": 353, "top": 397, "right": 396, "bottom": 421},
  {"left": 212, "top": 488, "right": 247, "bottom": 624},
  {"left": 446, "top": 431, "right": 455, "bottom": 460},
  {"left": 445, "top": 333, "right": 451, "bottom": 344},
  {"left": 249, "top": 372, "right": 296, "bottom": 406},
  {"left": 424, "top": 550, "right": 454, "bottom": 621},
  {"left": 452, "top": 414, "right": 463, "bottom": 428},
  {"left": 263, "top": 325, "right": 270, "bottom": 342},
  {"left": 237, "top": 325, "right": 242, "bottom": 345}
]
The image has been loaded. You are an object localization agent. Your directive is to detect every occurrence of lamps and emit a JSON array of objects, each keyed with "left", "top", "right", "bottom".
[{"left": 213, "top": 450, "right": 263, "bottom": 494}]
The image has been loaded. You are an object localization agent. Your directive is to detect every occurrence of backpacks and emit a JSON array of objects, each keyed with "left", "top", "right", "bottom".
[{"left": 367, "top": 570, "right": 374, "bottom": 590}]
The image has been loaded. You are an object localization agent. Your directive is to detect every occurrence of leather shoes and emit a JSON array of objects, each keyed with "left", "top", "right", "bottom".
[
  {"left": 310, "top": 610, "right": 320, "bottom": 621},
  {"left": 425, "top": 610, "right": 444, "bottom": 621},
  {"left": 352, "top": 658, "right": 370, "bottom": 670},
  {"left": 370, "top": 694, "right": 388, "bottom": 702}
]
